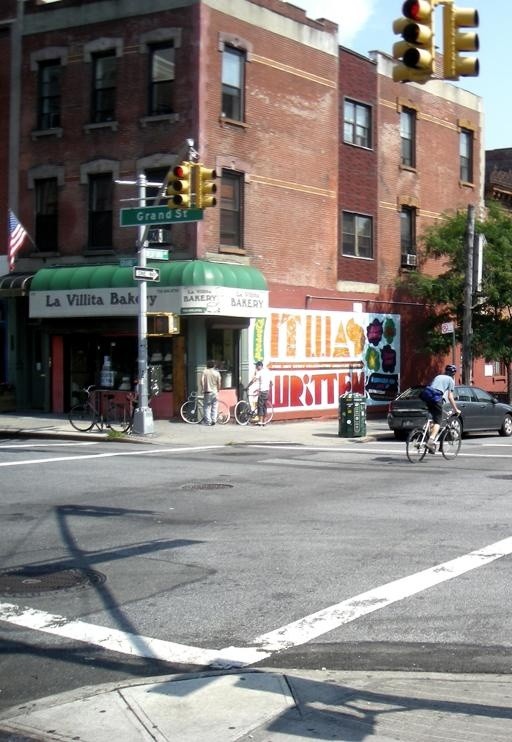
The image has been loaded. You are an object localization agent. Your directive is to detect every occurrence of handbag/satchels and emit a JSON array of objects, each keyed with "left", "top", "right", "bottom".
[{"left": 420, "top": 386, "right": 443, "bottom": 403}]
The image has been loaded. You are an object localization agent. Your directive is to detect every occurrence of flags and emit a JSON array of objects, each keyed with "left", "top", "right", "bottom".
[{"left": 7, "top": 208, "right": 28, "bottom": 272}]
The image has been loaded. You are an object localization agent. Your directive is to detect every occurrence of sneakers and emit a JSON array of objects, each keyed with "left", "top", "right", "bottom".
[
  {"left": 425, "top": 441, "right": 436, "bottom": 454},
  {"left": 255, "top": 421, "right": 266, "bottom": 427}
]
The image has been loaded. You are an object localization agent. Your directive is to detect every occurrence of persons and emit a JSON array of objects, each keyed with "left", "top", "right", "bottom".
[
  {"left": 422, "top": 364, "right": 461, "bottom": 455},
  {"left": 200, "top": 360, "right": 222, "bottom": 426},
  {"left": 243, "top": 361, "right": 274, "bottom": 427}
]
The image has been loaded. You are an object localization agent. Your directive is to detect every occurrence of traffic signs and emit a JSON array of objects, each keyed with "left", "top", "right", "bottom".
[
  {"left": 132, "top": 265, "right": 161, "bottom": 283},
  {"left": 145, "top": 247, "right": 169, "bottom": 262},
  {"left": 115, "top": 202, "right": 205, "bottom": 229}
]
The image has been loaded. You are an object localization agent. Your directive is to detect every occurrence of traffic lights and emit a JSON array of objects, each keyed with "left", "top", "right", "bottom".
[
  {"left": 173, "top": 162, "right": 192, "bottom": 210},
  {"left": 392, "top": 15, "right": 423, "bottom": 85},
  {"left": 194, "top": 162, "right": 218, "bottom": 210},
  {"left": 150, "top": 313, "right": 175, "bottom": 333},
  {"left": 166, "top": 166, "right": 179, "bottom": 211},
  {"left": 399, "top": 0, "right": 436, "bottom": 76},
  {"left": 441, "top": 2, "right": 481, "bottom": 82}
]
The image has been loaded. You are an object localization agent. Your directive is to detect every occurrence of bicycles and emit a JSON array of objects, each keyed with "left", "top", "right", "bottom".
[
  {"left": 67, "top": 382, "right": 133, "bottom": 433},
  {"left": 403, "top": 408, "right": 464, "bottom": 469},
  {"left": 233, "top": 388, "right": 275, "bottom": 426},
  {"left": 120, "top": 392, "right": 152, "bottom": 433},
  {"left": 178, "top": 390, "right": 232, "bottom": 427}
]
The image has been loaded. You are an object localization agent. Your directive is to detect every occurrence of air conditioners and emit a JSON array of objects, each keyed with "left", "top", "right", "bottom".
[{"left": 402, "top": 252, "right": 417, "bottom": 267}]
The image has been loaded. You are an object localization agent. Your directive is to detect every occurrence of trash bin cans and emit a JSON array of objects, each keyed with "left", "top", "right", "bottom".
[{"left": 338, "top": 392, "right": 367, "bottom": 437}]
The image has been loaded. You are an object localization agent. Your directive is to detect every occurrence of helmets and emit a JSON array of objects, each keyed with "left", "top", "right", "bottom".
[{"left": 445, "top": 363, "right": 457, "bottom": 375}]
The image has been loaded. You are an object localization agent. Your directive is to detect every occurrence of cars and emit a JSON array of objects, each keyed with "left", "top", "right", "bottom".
[{"left": 385, "top": 382, "right": 512, "bottom": 441}]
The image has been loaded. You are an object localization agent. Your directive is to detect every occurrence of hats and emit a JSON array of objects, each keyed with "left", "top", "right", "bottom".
[{"left": 253, "top": 361, "right": 263, "bottom": 366}]
